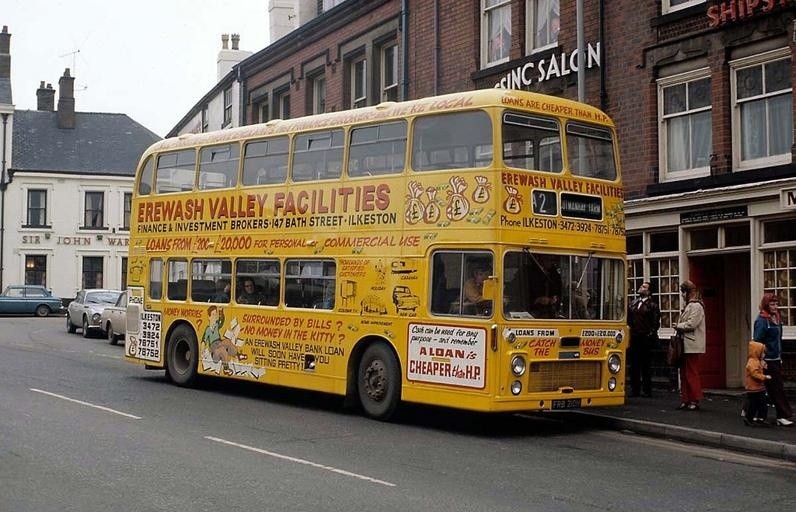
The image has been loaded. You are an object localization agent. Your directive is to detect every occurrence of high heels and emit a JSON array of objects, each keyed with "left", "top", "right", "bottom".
[{"left": 777, "top": 418, "right": 793, "bottom": 426}]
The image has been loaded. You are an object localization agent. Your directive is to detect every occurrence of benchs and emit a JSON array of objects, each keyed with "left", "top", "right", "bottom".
[
  {"left": 158, "top": 167, "right": 374, "bottom": 193},
  {"left": 150, "top": 277, "right": 324, "bottom": 309}
]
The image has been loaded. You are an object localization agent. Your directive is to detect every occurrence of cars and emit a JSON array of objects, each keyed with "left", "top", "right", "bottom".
[
  {"left": 0, "top": 284, "right": 64, "bottom": 317},
  {"left": 100, "top": 289, "right": 127, "bottom": 344},
  {"left": 66, "top": 288, "right": 123, "bottom": 338}
]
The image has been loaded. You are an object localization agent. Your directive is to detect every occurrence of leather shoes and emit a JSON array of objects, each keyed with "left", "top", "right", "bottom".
[
  {"left": 675, "top": 401, "right": 698, "bottom": 410},
  {"left": 627, "top": 391, "right": 651, "bottom": 398},
  {"left": 741, "top": 409, "right": 771, "bottom": 427}
]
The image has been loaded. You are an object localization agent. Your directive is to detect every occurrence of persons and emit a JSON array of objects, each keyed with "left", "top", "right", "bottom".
[
  {"left": 514, "top": 251, "right": 564, "bottom": 318},
  {"left": 564, "top": 281, "right": 589, "bottom": 319},
  {"left": 448, "top": 263, "right": 509, "bottom": 317},
  {"left": 213, "top": 278, "right": 231, "bottom": 304},
  {"left": 236, "top": 276, "right": 264, "bottom": 306},
  {"left": 671, "top": 279, "right": 708, "bottom": 411},
  {"left": 742, "top": 340, "right": 774, "bottom": 427},
  {"left": 739, "top": 293, "right": 794, "bottom": 427},
  {"left": 626, "top": 282, "right": 662, "bottom": 398}
]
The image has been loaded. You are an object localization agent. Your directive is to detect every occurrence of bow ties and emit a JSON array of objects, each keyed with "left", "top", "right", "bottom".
[{"left": 638, "top": 300, "right": 643, "bottom": 303}]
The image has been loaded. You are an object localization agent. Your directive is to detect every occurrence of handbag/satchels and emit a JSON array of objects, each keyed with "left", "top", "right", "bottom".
[{"left": 665, "top": 336, "right": 684, "bottom": 368}]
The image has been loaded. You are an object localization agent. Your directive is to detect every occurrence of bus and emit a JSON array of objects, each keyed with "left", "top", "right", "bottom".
[{"left": 124, "top": 89, "right": 630, "bottom": 422}]
